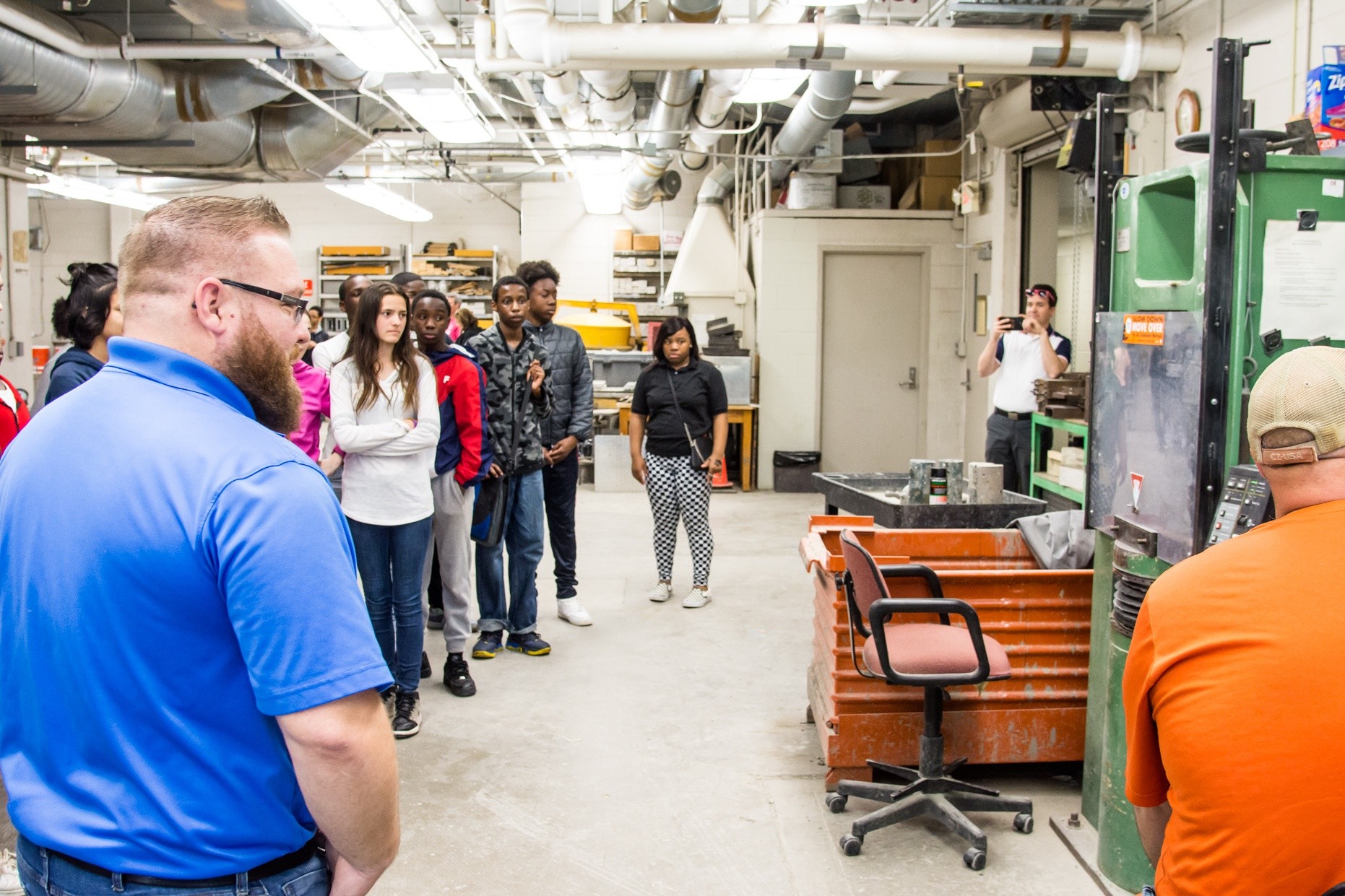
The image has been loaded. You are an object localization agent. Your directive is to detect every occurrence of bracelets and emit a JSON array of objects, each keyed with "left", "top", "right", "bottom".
[{"left": 407, "top": 418, "right": 417, "bottom": 428}]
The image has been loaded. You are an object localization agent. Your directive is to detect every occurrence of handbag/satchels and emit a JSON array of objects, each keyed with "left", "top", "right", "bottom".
[
  {"left": 689, "top": 434, "right": 714, "bottom": 472},
  {"left": 470, "top": 457, "right": 515, "bottom": 548}
]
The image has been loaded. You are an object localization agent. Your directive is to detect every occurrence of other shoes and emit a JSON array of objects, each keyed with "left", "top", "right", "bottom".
[{"left": 0, "top": 848, "right": 27, "bottom": 896}]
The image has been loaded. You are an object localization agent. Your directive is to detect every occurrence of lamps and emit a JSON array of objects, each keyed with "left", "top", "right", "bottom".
[
  {"left": 275, "top": 0, "right": 441, "bottom": 74},
  {"left": 322, "top": 176, "right": 435, "bottom": 223},
  {"left": 380, "top": 72, "right": 499, "bottom": 145}
]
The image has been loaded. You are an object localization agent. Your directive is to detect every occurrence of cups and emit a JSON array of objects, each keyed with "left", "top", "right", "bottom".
[{"left": 33, "top": 346, "right": 50, "bottom": 373}]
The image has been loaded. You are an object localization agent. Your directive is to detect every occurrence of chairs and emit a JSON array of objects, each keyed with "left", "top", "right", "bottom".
[{"left": 820, "top": 524, "right": 1038, "bottom": 870}]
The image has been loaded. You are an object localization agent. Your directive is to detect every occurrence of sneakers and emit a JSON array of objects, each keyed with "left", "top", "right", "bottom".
[
  {"left": 472, "top": 629, "right": 504, "bottom": 658},
  {"left": 682, "top": 587, "right": 712, "bottom": 607},
  {"left": 395, "top": 650, "right": 432, "bottom": 677},
  {"left": 391, "top": 691, "right": 423, "bottom": 738},
  {"left": 649, "top": 582, "right": 673, "bottom": 601},
  {"left": 426, "top": 607, "right": 478, "bottom": 632},
  {"left": 557, "top": 596, "right": 594, "bottom": 626},
  {"left": 378, "top": 685, "right": 398, "bottom": 721},
  {"left": 505, "top": 632, "right": 551, "bottom": 656},
  {"left": 443, "top": 654, "right": 476, "bottom": 697}
]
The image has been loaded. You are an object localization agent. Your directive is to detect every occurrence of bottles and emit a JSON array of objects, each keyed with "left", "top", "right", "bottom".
[{"left": 928, "top": 468, "right": 947, "bottom": 505}]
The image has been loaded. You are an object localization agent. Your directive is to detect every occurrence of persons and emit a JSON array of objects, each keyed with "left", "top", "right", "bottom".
[
  {"left": 1122, "top": 346, "right": 1345, "bottom": 896},
  {"left": 45, "top": 263, "right": 124, "bottom": 406},
  {"left": 629, "top": 316, "right": 728, "bottom": 608},
  {"left": 30, "top": 341, "right": 74, "bottom": 419},
  {"left": 0, "top": 253, "right": 32, "bottom": 459},
  {"left": 978, "top": 284, "right": 1072, "bottom": 499},
  {"left": 0, "top": 196, "right": 401, "bottom": 896},
  {"left": 285, "top": 261, "right": 597, "bottom": 737}
]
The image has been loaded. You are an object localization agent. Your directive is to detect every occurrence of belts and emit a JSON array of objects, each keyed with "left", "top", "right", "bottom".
[
  {"left": 994, "top": 407, "right": 1032, "bottom": 420},
  {"left": 44, "top": 833, "right": 328, "bottom": 889}
]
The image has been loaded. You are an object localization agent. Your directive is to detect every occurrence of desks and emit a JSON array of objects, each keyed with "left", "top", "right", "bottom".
[
  {"left": 612, "top": 400, "right": 760, "bottom": 494},
  {"left": 811, "top": 471, "right": 1047, "bottom": 532}
]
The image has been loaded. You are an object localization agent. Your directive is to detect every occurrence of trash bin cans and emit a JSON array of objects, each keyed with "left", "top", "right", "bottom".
[{"left": 774, "top": 451, "right": 820, "bottom": 493}]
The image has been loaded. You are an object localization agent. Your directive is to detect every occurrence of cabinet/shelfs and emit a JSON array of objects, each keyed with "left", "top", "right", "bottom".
[
  {"left": 405, "top": 243, "right": 500, "bottom": 324},
  {"left": 613, "top": 249, "right": 680, "bottom": 323},
  {"left": 314, "top": 243, "right": 405, "bottom": 339},
  {"left": 1027, "top": 412, "right": 1088, "bottom": 511}
]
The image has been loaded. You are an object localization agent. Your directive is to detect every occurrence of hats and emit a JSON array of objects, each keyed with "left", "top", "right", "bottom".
[{"left": 1246, "top": 345, "right": 1345, "bottom": 465}]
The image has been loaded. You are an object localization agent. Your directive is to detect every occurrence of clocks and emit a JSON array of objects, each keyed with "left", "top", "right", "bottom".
[{"left": 1174, "top": 87, "right": 1201, "bottom": 134}]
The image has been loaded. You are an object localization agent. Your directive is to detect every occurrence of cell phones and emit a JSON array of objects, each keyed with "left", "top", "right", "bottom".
[{"left": 999, "top": 316, "right": 1025, "bottom": 330}]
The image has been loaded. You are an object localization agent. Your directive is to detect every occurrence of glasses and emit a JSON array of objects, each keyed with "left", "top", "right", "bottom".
[
  {"left": 192, "top": 278, "right": 310, "bottom": 327},
  {"left": 1025, "top": 288, "right": 1055, "bottom": 302}
]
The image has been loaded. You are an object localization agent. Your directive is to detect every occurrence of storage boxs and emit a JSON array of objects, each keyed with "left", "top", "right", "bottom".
[
  {"left": 633, "top": 233, "right": 660, "bottom": 251},
  {"left": 613, "top": 228, "right": 633, "bottom": 250},
  {"left": 799, "top": 505, "right": 1087, "bottom": 784},
  {"left": 1303, "top": 63, "right": 1345, "bottom": 150},
  {"left": 662, "top": 229, "right": 684, "bottom": 250}
]
